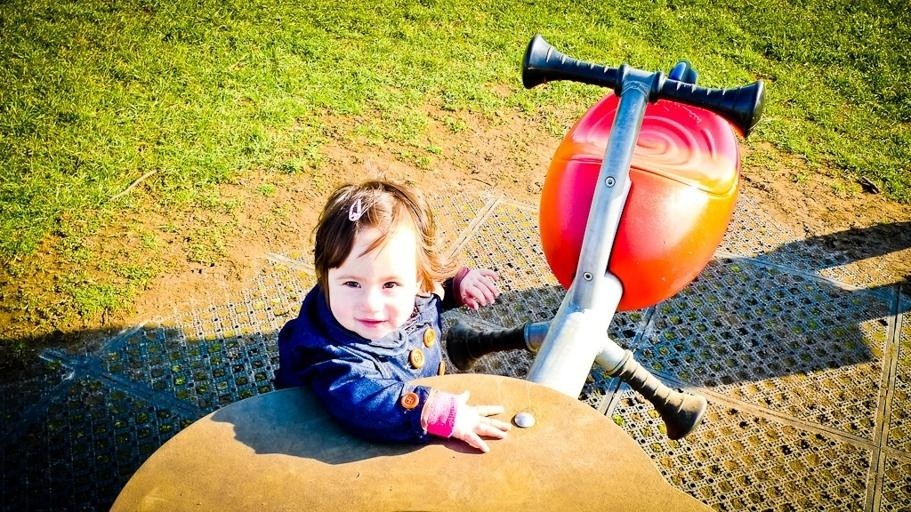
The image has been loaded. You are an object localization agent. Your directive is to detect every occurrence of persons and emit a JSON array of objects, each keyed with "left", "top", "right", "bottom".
[{"left": 273, "top": 176, "right": 511, "bottom": 454}]
